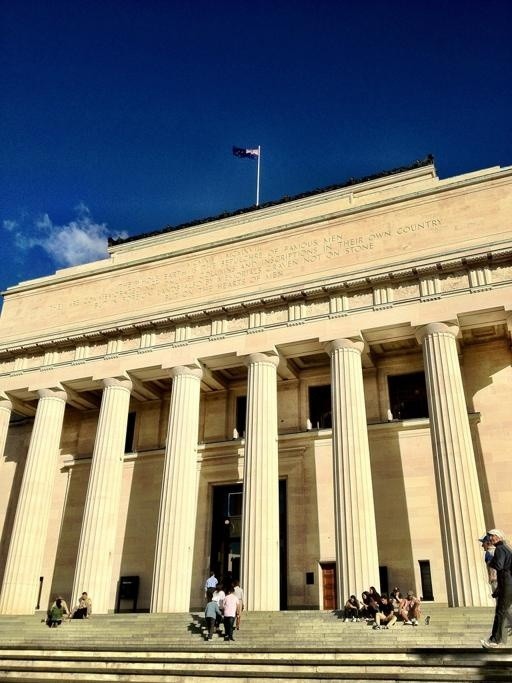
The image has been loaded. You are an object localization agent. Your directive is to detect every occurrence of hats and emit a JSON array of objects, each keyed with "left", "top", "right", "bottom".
[
  {"left": 479, "top": 536, "right": 490, "bottom": 542},
  {"left": 407, "top": 591, "right": 414, "bottom": 595},
  {"left": 487, "top": 529, "right": 505, "bottom": 538}
]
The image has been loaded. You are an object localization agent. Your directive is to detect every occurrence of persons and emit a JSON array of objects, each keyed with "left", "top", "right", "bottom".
[
  {"left": 342, "top": 584, "right": 422, "bottom": 629},
  {"left": 46, "top": 591, "right": 91, "bottom": 627},
  {"left": 204, "top": 571, "right": 244, "bottom": 640}
]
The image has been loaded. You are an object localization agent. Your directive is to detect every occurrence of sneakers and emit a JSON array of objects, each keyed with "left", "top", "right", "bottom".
[
  {"left": 481, "top": 640, "right": 503, "bottom": 648},
  {"left": 405, "top": 620, "right": 418, "bottom": 626}
]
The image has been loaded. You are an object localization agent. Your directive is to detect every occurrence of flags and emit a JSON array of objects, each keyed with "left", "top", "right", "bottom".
[{"left": 231, "top": 146, "right": 261, "bottom": 160}]
